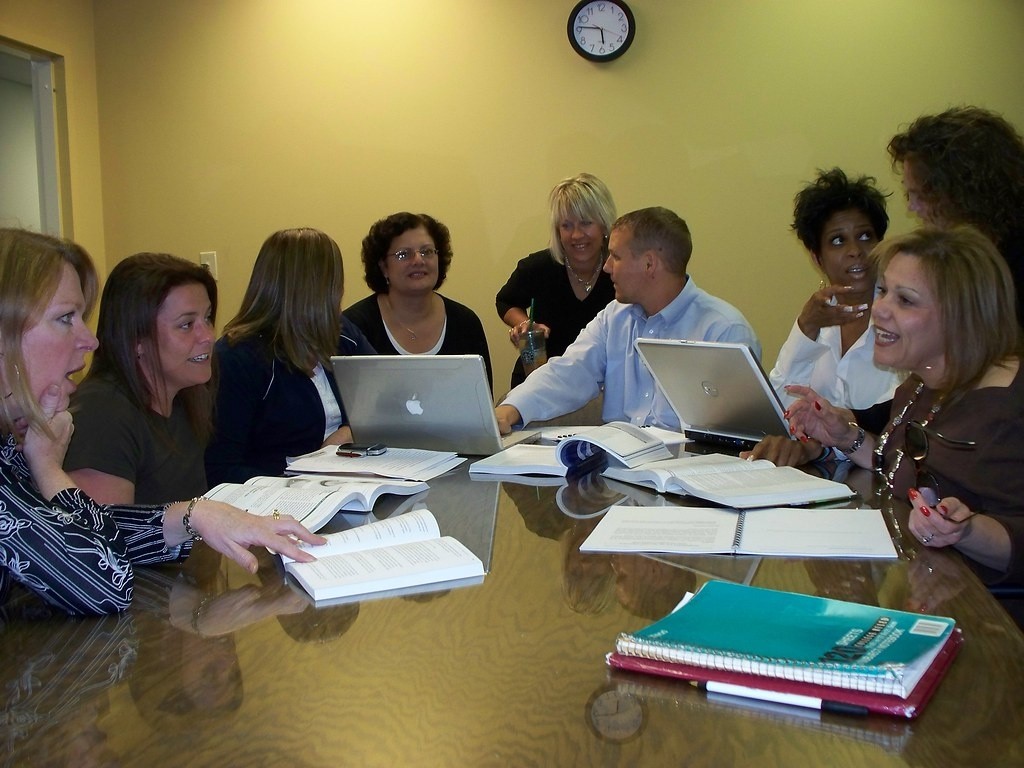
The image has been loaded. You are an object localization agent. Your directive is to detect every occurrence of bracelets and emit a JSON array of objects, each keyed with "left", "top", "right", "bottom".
[
  {"left": 190, "top": 586, "right": 211, "bottom": 639},
  {"left": 838, "top": 423, "right": 866, "bottom": 455},
  {"left": 182, "top": 496, "right": 213, "bottom": 542}
]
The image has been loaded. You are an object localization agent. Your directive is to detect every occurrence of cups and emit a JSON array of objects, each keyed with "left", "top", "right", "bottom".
[{"left": 517, "top": 330, "right": 548, "bottom": 377}]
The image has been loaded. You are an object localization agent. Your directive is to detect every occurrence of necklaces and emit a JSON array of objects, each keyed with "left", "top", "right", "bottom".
[
  {"left": 564, "top": 250, "right": 605, "bottom": 295},
  {"left": 881, "top": 496, "right": 923, "bottom": 563},
  {"left": 866, "top": 366, "right": 955, "bottom": 573},
  {"left": 385, "top": 294, "right": 437, "bottom": 345}
]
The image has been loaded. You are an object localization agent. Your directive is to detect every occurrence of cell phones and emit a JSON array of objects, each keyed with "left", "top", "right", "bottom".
[{"left": 336, "top": 443, "right": 387, "bottom": 456}]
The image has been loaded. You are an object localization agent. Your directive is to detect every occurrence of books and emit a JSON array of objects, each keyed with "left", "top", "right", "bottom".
[
  {"left": 200, "top": 472, "right": 431, "bottom": 555},
  {"left": 471, "top": 471, "right": 630, "bottom": 520},
  {"left": 578, "top": 500, "right": 902, "bottom": 560},
  {"left": 469, "top": 420, "right": 675, "bottom": 480},
  {"left": 608, "top": 576, "right": 968, "bottom": 720},
  {"left": 266, "top": 508, "right": 488, "bottom": 600},
  {"left": 603, "top": 452, "right": 861, "bottom": 511},
  {"left": 517, "top": 421, "right": 695, "bottom": 449}
]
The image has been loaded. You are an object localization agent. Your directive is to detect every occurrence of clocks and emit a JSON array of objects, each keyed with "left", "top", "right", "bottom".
[
  {"left": 584, "top": 683, "right": 649, "bottom": 746},
  {"left": 566, "top": 0, "right": 636, "bottom": 64}
]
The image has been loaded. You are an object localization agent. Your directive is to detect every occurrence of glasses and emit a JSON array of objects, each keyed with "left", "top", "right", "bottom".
[
  {"left": 389, "top": 247, "right": 439, "bottom": 261},
  {"left": 903, "top": 418, "right": 978, "bottom": 523}
]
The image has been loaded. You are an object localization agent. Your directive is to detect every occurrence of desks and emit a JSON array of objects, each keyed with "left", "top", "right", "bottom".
[{"left": 0, "top": 438, "right": 1024, "bottom": 768}]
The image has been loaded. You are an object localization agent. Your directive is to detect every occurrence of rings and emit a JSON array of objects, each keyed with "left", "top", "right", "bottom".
[
  {"left": 919, "top": 532, "right": 934, "bottom": 544},
  {"left": 919, "top": 558, "right": 937, "bottom": 575},
  {"left": 273, "top": 509, "right": 280, "bottom": 520}
]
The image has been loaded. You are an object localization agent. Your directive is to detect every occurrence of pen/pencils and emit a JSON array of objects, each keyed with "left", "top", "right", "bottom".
[{"left": 688, "top": 679, "right": 870, "bottom": 719}]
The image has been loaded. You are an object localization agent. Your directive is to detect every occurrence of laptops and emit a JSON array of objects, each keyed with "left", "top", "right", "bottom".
[
  {"left": 633, "top": 337, "right": 798, "bottom": 451},
  {"left": 330, "top": 354, "right": 542, "bottom": 455}
]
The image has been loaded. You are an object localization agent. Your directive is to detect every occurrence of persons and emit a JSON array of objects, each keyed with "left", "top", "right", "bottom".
[
  {"left": 769, "top": 102, "right": 1024, "bottom": 618},
  {"left": 491, "top": 208, "right": 762, "bottom": 438},
  {"left": 500, "top": 478, "right": 698, "bottom": 622},
  {"left": 0, "top": 228, "right": 379, "bottom": 617},
  {"left": 802, "top": 496, "right": 973, "bottom": 616},
  {"left": 0, "top": 535, "right": 360, "bottom": 768},
  {"left": 344, "top": 212, "right": 495, "bottom": 406},
  {"left": 497, "top": 173, "right": 620, "bottom": 391}
]
